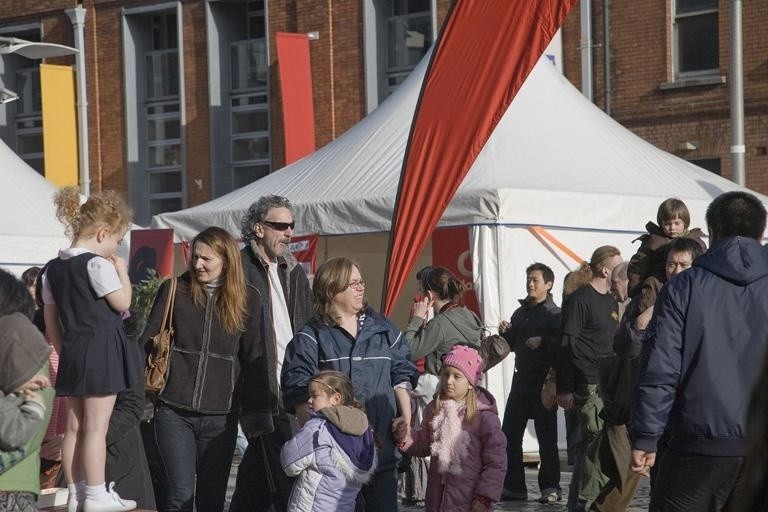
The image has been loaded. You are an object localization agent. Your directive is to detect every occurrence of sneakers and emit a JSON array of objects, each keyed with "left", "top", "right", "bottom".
[
  {"left": 540, "top": 488, "right": 562, "bottom": 502},
  {"left": 68, "top": 481, "right": 137, "bottom": 512},
  {"left": 501, "top": 489, "right": 527, "bottom": 500}
]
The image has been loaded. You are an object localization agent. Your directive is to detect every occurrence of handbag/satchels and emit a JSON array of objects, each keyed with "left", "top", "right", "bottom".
[
  {"left": 144, "top": 329, "right": 174, "bottom": 392},
  {"left": 482, "top": 334, "right": 510, "bottom": 372},
  {"left": 540, "top": 368, "right": 559, "bottom": 410}
]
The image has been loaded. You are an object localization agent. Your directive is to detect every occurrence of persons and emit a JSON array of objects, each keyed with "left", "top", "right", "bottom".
[{"left": 1, "top": 191, "right": 766, "bottom": 511}]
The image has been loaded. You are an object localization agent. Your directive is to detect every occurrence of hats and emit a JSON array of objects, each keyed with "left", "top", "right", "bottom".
[{"left": 444, "top": 345, "right": 485, "bottom": 388}]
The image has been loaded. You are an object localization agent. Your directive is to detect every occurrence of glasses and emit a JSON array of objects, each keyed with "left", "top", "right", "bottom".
[{"left": 265, "top": 220, "right": 295, "bottom": 230}]
